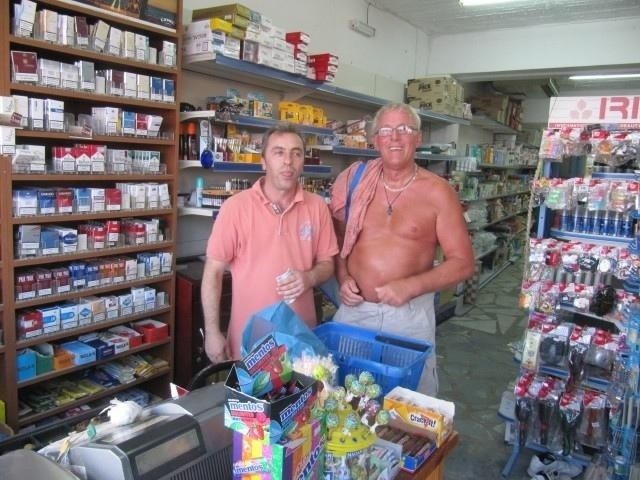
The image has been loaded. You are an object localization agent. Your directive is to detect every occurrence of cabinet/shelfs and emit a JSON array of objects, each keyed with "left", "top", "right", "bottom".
[
  {"left": 2, "top": 0, "right": 181, "bottom": 440},
  {"left": 504, "top": 96, "right": 640, "bottom": 477},
  {"left": 181, "top": 53, "right": 472, "bottom": 385},
  {"left": 469, "top": 110, "right": 538, "bottom": 298}
]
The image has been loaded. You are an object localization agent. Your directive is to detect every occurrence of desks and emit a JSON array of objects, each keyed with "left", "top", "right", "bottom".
[{"left": 394, "top": 391, "right": 459, "bottom": 479}]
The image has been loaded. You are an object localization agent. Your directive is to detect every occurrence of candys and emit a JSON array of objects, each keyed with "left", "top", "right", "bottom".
[{"left": 311, "top": 365, "right": 391, "bottom": 443}]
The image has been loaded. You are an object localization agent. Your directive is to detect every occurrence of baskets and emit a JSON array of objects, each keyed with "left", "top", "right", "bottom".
[{"left": 291, "top": 322, "right": 435, "bottom": 404}]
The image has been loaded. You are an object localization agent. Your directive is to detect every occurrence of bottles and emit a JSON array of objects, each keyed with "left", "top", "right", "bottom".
[
  {"left": 196, "top": 176, "right": 204, "bottom": 207},
  {"left": 179, "top": 124, "right": 184, "bottom": 159},
  {"left": 559, "top": 201, "right": 632, "bottom": 240},
  {"left": 189, "top": 122, "right": 197, "bottom": 160}
]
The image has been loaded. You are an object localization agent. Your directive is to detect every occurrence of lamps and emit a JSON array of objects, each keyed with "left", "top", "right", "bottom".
[{"left": 351, "top": 18, "right": 376, "bottom": 37}]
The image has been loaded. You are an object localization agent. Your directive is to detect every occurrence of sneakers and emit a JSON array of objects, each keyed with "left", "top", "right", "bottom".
[
  {"left": 526, "top": 451, "right": 583, "bottom": 478},
  {"left": 530, "top": 470, "right": 571, "bottom": 480}
]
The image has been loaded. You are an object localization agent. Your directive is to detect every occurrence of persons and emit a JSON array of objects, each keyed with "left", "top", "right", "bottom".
[
  {"left": 327, "top": 98, "right": 475, "bottom": 398},
  {"left": 200, "top": 121, "right": 337, "bottom": 384}
]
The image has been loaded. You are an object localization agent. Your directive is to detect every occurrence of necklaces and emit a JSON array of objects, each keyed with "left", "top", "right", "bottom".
[
  {"left": 382, "top": 169, "right": 416, "bottom": 217},
  {"left": 380, "top": 164, "right": 419, "bottom": 192},
  {"left": 260, "top": 184, "right": 295, "bottom": 216}
]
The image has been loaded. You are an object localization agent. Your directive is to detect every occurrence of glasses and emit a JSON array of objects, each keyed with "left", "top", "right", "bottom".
[{"left": 375, "top": 125, "right": 419, "bottom": 138}]
